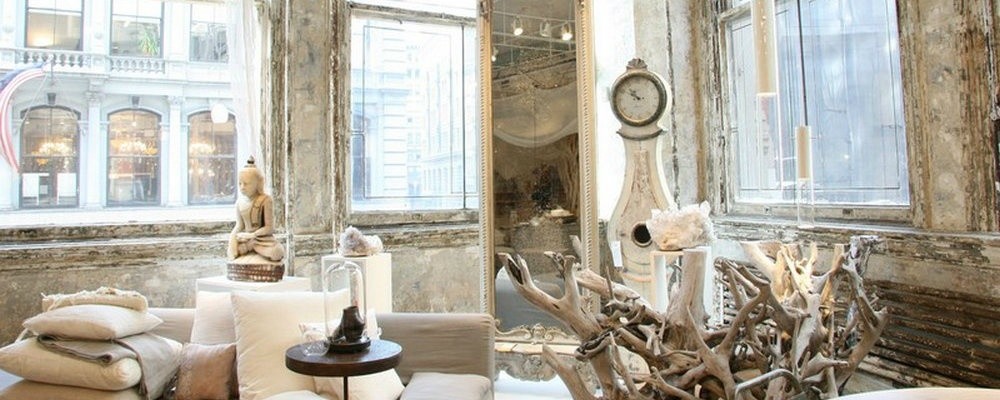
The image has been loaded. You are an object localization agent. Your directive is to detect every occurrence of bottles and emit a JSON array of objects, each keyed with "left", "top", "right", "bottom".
[{"left": 321, "top": 252, "right": 371, "bottom": 352}]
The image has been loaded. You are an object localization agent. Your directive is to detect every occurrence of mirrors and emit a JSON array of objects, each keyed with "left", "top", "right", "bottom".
[{"left": 479, "top": 0, "right": 598, "bottom": 356}]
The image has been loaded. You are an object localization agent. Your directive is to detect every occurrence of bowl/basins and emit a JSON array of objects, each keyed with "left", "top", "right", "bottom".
[{"left": 545, "top": 214, "right": 577, "bottom": 223}]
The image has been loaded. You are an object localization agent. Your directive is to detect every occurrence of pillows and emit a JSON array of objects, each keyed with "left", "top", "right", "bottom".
[{"left": 0, "top": 289, "right": 406, "bottom": 400}]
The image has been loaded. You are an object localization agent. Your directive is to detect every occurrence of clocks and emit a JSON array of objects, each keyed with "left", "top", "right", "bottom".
[{"left": 607, "top": 58, "right": 676, "bottom": 290}]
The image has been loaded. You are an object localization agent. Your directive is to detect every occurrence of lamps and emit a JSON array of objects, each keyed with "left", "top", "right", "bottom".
[
  {"left": 795, "top": 0, "right": 814, "bottom": 229},
  {"left": 540, "top": 22, "right": 549, "bottom": 36},
  {"left": 562, "top": 23, "right": 573, "bottom": 40},
  {"left": 750, "top": 0, "right": 783, "bottom": 192},
  {"left": 514, "top": 19, "right": 523, "bottom": 34}
]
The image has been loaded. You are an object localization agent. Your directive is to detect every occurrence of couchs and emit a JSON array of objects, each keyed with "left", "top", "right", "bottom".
[{"left": 0, "top": 309, "right": 495, "bottom": 400}]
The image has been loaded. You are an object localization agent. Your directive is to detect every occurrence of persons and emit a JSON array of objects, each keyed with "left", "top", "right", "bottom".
[{"left": 226, "top": 154, "right": 285, "bottom": 262}]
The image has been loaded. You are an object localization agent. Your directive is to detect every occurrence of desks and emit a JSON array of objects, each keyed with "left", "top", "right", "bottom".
[{"left": 197, "top": 275, "right": 310, "bottom": 292}]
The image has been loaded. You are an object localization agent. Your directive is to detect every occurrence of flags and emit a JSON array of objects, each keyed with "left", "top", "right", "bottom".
[{"left": 0, "top": 64, "right": 47, "bottom": 173}]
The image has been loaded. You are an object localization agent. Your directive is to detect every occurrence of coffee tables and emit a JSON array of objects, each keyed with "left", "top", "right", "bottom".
[{"left": 286, "top": 339, "right": 402, "bottom": 400}]
